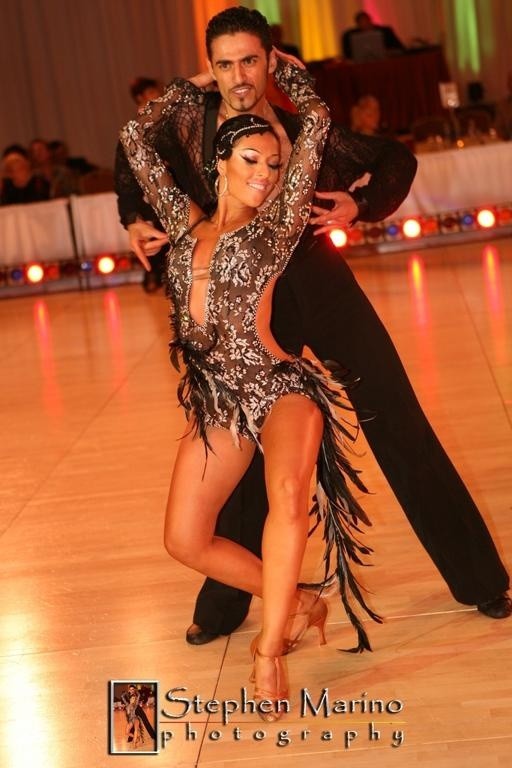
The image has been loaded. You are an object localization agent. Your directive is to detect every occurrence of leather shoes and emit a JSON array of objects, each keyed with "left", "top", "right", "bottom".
[
  {"left": 186, "top": 622, "right": 221, "bottom": 644},
  {"left": 476, "top": 590, "right": 512, "bottom": 618}
]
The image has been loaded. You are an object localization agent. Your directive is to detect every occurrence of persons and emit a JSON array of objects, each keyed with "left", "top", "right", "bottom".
[
  {"left": 344, "top": 13, "right": 400, "bottom": 57},
  {"left": 113, "top": 6, "right": 511, "bottom": 644},
  {"left": 461, "top": 81, "right": 495, "bottom": 122},
  {"left": 270, "top": 23, "right": 301, "bottom": 57},
  {"left": 117, "top": 42, "right": 333, "bottom": 723},
  {"left": 3, "top": 74, "right": 383, "bottom": 294}
]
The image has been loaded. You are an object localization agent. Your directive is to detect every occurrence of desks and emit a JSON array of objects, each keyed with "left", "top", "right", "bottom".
[{"left": 0, "top": 49, "right": 512, "bottom": 289}]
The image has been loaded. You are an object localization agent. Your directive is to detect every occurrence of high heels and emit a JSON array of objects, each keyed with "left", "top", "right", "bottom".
[
  {"left": 251, "top": 595, "right": 328, "bottom": 655},
  {"left": 248, "top": 641, "right": 290, "bottom": 723}
]
